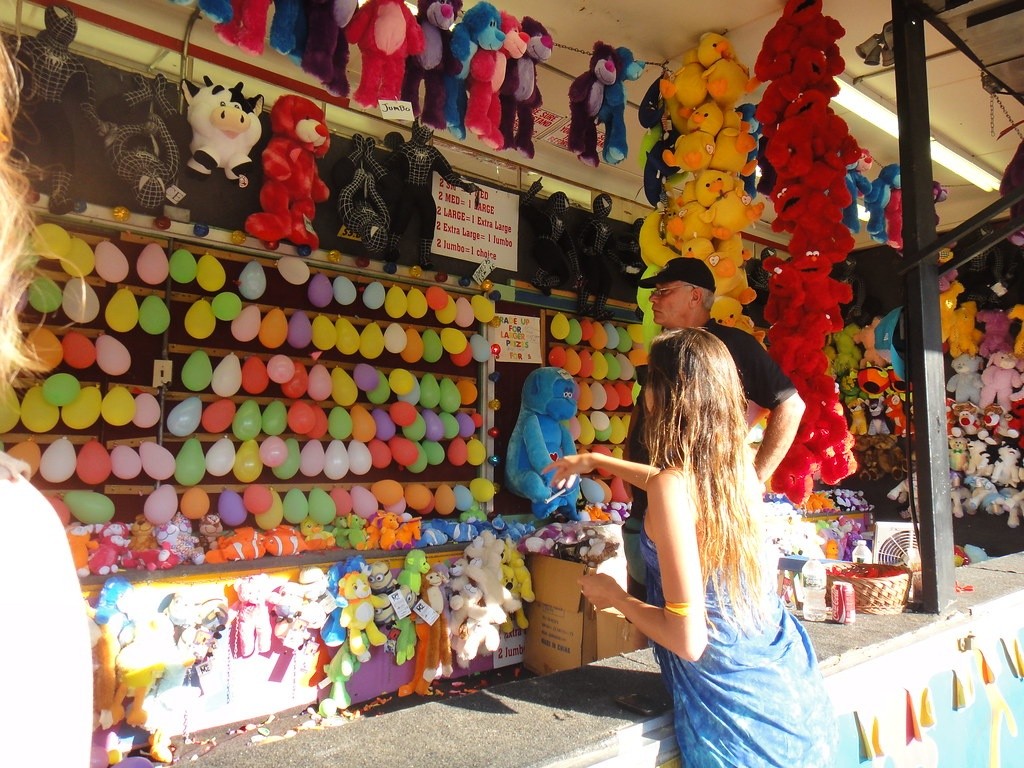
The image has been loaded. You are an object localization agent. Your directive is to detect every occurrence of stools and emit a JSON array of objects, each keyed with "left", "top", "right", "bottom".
[{"left": 778, "top": 555, "right": 852, "bottom": 611}]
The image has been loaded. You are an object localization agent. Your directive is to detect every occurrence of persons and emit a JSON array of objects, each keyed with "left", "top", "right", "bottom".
[
  {"left": 541, "top": 328, "right": 838, "bottom": 768},
  {"left": 0, "top": 39, "right": 95, "bottom": 768},
  {"left": 620, "top": 256, "right": 806, "bottom": 601}
]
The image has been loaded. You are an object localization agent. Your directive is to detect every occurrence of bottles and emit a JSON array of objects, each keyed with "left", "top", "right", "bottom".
[
  {"left": 853, "top": 539, "right": 872, "bottom": 564},
  {"left": 801, "top": 556, "right": 828, "bottom": 621}
]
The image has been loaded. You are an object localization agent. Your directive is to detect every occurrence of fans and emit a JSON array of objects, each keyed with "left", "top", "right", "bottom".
[{"left": 872, "top": 522, "right": 920, "bottom": 567}]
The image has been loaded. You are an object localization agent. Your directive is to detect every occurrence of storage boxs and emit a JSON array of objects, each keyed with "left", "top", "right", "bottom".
[
  {"left": 521, "top": 554, "right": 596, "bottom": 674},
  {"left": 592, "top": 558, "right": 648, "bottom": 660}
]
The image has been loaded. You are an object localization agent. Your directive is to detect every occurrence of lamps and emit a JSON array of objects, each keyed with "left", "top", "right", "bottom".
[{"left": 830, "top": 72, "right": 1003, "bottom": 193}]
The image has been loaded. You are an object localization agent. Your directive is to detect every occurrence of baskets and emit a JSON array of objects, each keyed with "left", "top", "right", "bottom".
[{"left": 824, "top": 563, "right": 913, "bottom": 614}]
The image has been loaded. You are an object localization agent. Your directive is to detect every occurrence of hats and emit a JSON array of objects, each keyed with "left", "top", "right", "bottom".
[{"left": 638, "top": 257, "right": 716, "bottom": 294}]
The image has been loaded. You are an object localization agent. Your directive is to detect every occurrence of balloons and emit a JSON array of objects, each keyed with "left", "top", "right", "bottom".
[
  {"left": 549, "top": 312, "right": 648, "bottom": 505},
  {"left": 0, "top": 222, "right": 495, "bottom": 530}
]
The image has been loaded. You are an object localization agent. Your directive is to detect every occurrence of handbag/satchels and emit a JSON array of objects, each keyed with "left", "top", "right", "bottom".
[{"left": 621, "top": 516, "right": 647, "bottom": 585}]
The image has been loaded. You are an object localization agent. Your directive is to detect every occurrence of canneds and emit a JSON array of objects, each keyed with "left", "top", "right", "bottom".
[{"left": 831, "top": 582, "right": 855, "bottom": 625}]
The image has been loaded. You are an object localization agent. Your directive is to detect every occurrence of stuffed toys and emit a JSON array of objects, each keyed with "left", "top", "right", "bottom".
[
  {"left": 632, "top": 0, "right": 1024, "bottom": 561},
  {"left": 506, "top": 367, "right": 582, "bottom": 521},
  {"left": 10, "top": 0, "right": 646, "bottom": 321},
  {"left": 67, "top": 502, "right": 633, "bottom": 764}
]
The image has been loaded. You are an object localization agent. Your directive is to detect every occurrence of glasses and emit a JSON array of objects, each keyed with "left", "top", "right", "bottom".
[
  {"left": 635, "top": 364, "right": 649, "bottom": 386},
  {"left": 651, "top": 284, "right": 695, "bottom": 297}
]
